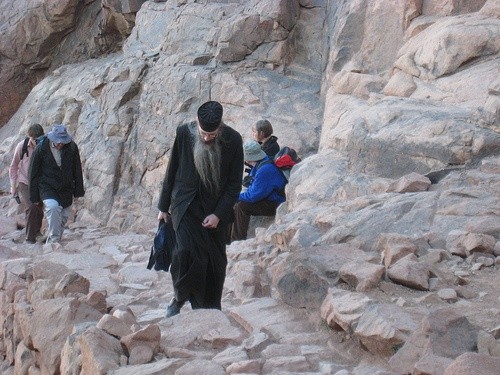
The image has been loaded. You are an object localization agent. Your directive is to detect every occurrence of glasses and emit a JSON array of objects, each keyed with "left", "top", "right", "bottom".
[{"left": 198, "top": 131, "right": 221, "bottom": 138}]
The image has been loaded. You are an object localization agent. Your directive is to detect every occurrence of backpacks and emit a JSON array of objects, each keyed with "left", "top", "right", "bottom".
[{"left": 256, "top": 145, "right": 302, "bottom": 177}]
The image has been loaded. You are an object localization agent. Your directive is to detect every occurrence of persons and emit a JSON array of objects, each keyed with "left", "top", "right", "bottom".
[
  {"left": 229, "top": 139, "right": 287, "bottom": 242},
  {"left": 157, "top": 101, "right": 244, "bottom": 317},
  {"left": 29, "top": 124, "right": 85, "bottom": 245},
  {"left": 252, "top": 120, "right": 280, "bottom": 157},
  {"left": 9, "top": 123, "right": 44, "bottom": 244}
]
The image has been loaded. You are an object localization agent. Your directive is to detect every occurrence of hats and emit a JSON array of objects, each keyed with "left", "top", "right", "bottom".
[
  {"left": 197, "top": 101, "right": 223, "bottom": 132},
  {"left": 242, "top": 139, "right": 267, "bottom": 161},
  {"left": 48, "top": 125, "right": 73, "bottom": 144}
]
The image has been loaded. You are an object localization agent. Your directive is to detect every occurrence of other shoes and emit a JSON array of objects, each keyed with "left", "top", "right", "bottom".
[
  {"left": 166, "top": 295, "right": 186, "bottom": 318},
  {"left": 50, "top": 234, "right": 60, "bottom": 242},
  {"left": 46, "top": 236, "right": 53, "bottom": 244},
  {"left": 26, "top": 237, "right": 36, "bottom": 244}
]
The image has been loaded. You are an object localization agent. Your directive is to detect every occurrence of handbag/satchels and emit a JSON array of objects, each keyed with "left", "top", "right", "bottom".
[{"left": 147, "top": 213, "right": 176, "bottom": 272}]
